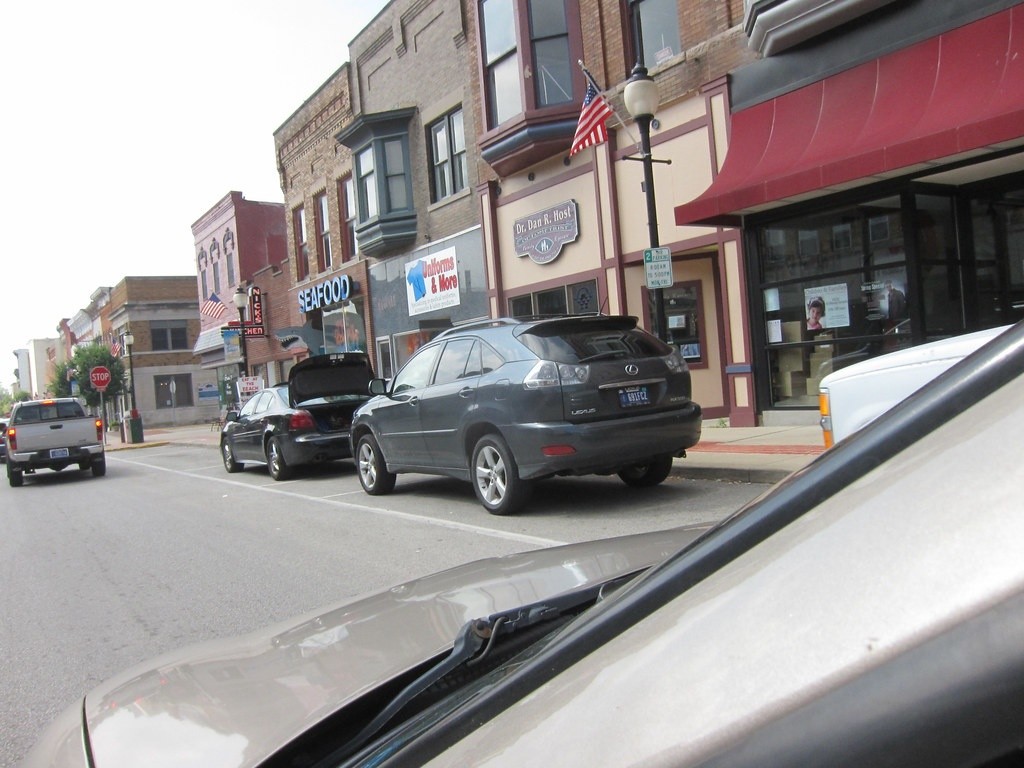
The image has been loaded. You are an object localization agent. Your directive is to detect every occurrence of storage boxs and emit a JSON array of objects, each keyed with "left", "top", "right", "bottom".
[{"left": 775, "top": 321, "right": 834, "bottom": 397}]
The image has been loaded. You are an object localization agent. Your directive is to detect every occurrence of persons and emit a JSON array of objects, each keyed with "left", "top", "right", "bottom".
[
  {"left": 884, "top": 281, "right": 906, "bottom": 320},
  {"left": 807, "top": 296, "right": 826, "bottom": 330}
]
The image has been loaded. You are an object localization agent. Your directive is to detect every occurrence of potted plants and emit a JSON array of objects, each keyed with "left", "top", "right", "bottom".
[{"left": 112, "top": 421, "right": 119, "bottom": 431}]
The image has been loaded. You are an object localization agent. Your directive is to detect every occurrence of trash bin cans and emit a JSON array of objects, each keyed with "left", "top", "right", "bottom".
[{"left": 125, "top": 418, "right": 144, "bottom": 444}]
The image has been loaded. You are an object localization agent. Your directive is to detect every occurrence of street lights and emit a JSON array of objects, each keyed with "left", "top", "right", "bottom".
[
  {"left": 233, "top": 284, "right": 251, "bottom": 396},
  {"left": 124, "top": 330, "right": 138, "bottom": 418},
  {"left": 624, "top": 53, "right": 668, "bottom": 344}
]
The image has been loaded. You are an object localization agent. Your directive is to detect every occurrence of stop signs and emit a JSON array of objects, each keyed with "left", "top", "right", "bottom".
[{"left": 90, "top": 367, "right": 111, "bottom": 387}]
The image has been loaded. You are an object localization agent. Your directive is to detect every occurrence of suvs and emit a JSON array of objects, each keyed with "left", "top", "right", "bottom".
[{"left": 349, "top": 313, "right": 702, "bottom": 517}]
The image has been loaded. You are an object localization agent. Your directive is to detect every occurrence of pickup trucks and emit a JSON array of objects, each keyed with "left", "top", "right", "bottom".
[{"left": 5, "top": 398, "right": 107, "bottom": 488}]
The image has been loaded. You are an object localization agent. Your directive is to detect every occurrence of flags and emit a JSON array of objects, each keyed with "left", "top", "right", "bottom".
[
  {"left": 568, "top": 70, "right": 616, "bottom": 156},
  {"left": 200, "top": 293, "right": 228, "bottom": 320},
  {"left": 110, "top": 335, "right": 122, "bottom": 357},
  {"left": 66, "top": 366, "right": 74, "bottom": 382}
]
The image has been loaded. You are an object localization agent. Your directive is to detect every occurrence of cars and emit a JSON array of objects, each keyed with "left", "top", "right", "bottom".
[
  {"left": 220, "top": 353, "right": 376, "bottom": 482},
  {"left": 0, "top": 418, "right": 10, "bottom": 460}
]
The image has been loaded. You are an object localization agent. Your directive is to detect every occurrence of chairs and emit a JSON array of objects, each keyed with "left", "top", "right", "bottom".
[{"left": 211, "top": 410, "right": 240, "bottom": 432}]
